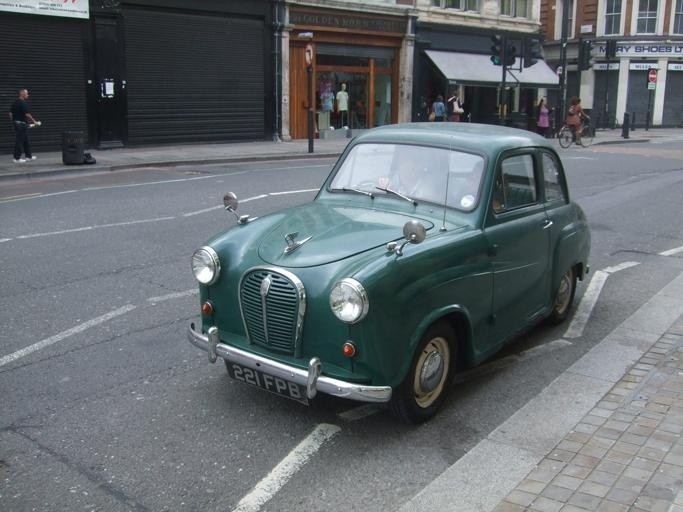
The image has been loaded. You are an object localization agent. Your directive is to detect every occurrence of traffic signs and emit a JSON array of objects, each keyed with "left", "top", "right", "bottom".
[{"left": 648, "top": 83, "right": 656, "bottom": 89}]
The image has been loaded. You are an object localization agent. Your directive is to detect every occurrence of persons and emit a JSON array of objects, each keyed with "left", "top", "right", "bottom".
[
  {"left": 535, "top": 96, "right": 556, "bottom": 137},
  {"left": 337, "top": 82, "right": 350, "bottom": 114},
  {"left": 321, "top": 84, "right": 334, "bottom": 113},
  {"left": 447, "top": 90, "right": 465, "bottom": 122},
  {"left": 431, "top": 95, "right": 446, "bottom": 122},
  {"left": 565, "top": 97, "right": 589, "bottom": 142},
  {"left": 9, "top": 89, "right": 39, "bottom": 163}
]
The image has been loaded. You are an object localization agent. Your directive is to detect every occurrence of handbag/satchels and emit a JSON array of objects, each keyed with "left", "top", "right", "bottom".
[
  {"left": 452, "top": 98, "right": 464, "bottom": 113},
  {"left": 427, "top": 112, "right": 434, "bottom": 121}
]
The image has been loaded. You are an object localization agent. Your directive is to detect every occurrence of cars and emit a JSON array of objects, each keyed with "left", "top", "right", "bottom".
[{"left": 187, "top": 119, "right": 591, "bottom": 426}]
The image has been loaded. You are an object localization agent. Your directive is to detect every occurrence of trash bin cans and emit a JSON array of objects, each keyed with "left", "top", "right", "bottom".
[{"left": 62, "top": 130, "right": 87, "bottom": 165}]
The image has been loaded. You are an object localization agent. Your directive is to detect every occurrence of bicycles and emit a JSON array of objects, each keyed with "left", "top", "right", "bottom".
[{"left": 557, "top": 116, "right": 595, "bottom": 148}]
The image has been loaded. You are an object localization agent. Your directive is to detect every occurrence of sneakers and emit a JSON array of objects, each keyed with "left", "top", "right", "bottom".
[
  {"left": 12, "top": 159, "right": 26, "bottom": 163},
  {"left": 25, "top": 155, "right": 36, "bottom": 162}
]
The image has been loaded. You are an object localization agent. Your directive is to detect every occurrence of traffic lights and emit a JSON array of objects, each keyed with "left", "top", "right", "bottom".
[
  {"left": 607, "top": 39, "right": 617, "bottom": 60},
  {"left": 578, "top": 38, "right": 593, "bottom": 70},
  {"left": 525, "top": 36, "right": 539, "bottom": 67},
  {"left": 508, "top": 34, "right": 516, "bottom": 66},
  {"left": 491, "top": 33, "right": 503, "bottom": 65}
]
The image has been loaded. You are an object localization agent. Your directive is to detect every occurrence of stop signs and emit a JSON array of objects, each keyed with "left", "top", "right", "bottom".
[{"left": 647, "top": 69, "right": 657, "bottom": 82}]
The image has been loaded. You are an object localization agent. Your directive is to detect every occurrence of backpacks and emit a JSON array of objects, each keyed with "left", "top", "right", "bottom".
[{"left": 567, "top": 105, "right": 577, "bottom": 115}]
